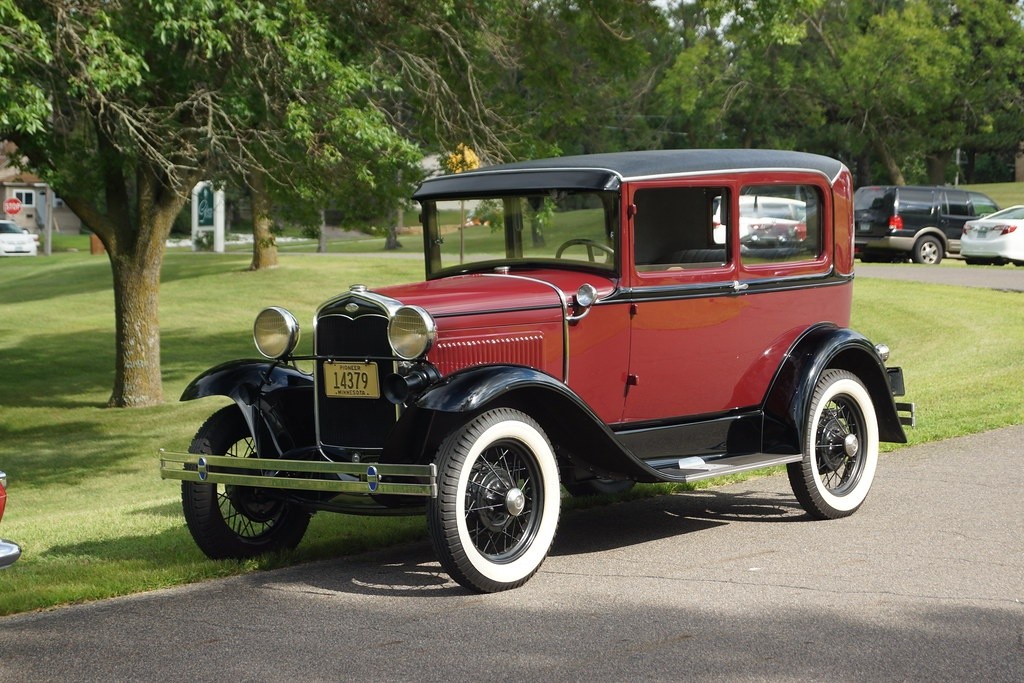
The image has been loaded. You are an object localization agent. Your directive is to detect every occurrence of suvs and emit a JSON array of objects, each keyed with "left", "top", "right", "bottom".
[{"left": 162, "top": 149, "right": 913, "bottom": 593}]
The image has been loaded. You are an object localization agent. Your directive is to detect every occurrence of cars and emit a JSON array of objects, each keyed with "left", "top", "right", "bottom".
[
  {"left": 0, "top": 221, "right": 39, "bottom": 256},
  {"left": 960, "top": 205, "right": 1024, "bottom": 267}
]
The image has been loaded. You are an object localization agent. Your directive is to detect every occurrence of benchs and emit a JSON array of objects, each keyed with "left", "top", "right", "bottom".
[{"left": 655, "top": 247, "right": 816, "bottom": 270}]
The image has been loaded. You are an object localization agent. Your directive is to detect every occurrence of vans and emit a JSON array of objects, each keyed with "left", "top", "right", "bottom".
[
  {"left": 712, "top": 194, "right": 807, "bottom": 245},
  {"left": 854, "top": 186, "right": 1003, "bottom": 265}
]
